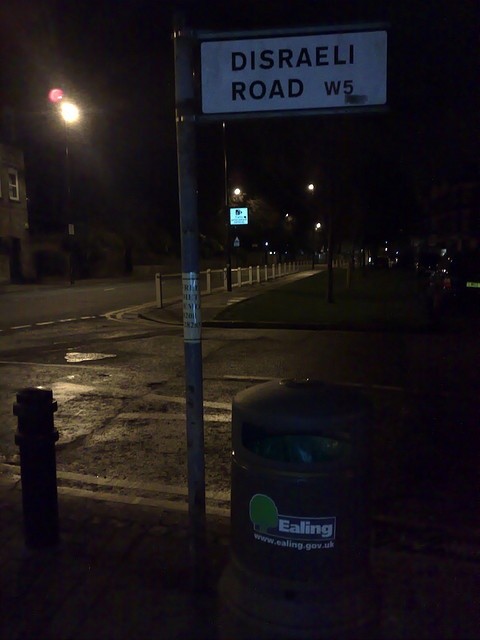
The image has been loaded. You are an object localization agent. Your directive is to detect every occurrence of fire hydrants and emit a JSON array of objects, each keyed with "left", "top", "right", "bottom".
[{"left": 9, "top": 385, "right": 74, "bottom": 526}]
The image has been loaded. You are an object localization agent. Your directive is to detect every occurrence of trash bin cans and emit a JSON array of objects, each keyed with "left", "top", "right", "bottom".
[{"left": 230, "top": 376, "right": 379, "bottom": 600}]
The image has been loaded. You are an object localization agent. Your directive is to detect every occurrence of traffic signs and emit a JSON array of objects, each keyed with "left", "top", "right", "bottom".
[{"left": 197, "top": 30, "right": 389, "bottom": 115}]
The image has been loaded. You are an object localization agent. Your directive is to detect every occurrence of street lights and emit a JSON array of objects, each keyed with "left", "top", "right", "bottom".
[{"left": 59, "top": 100, "right": 80, "bottom": 285}]
